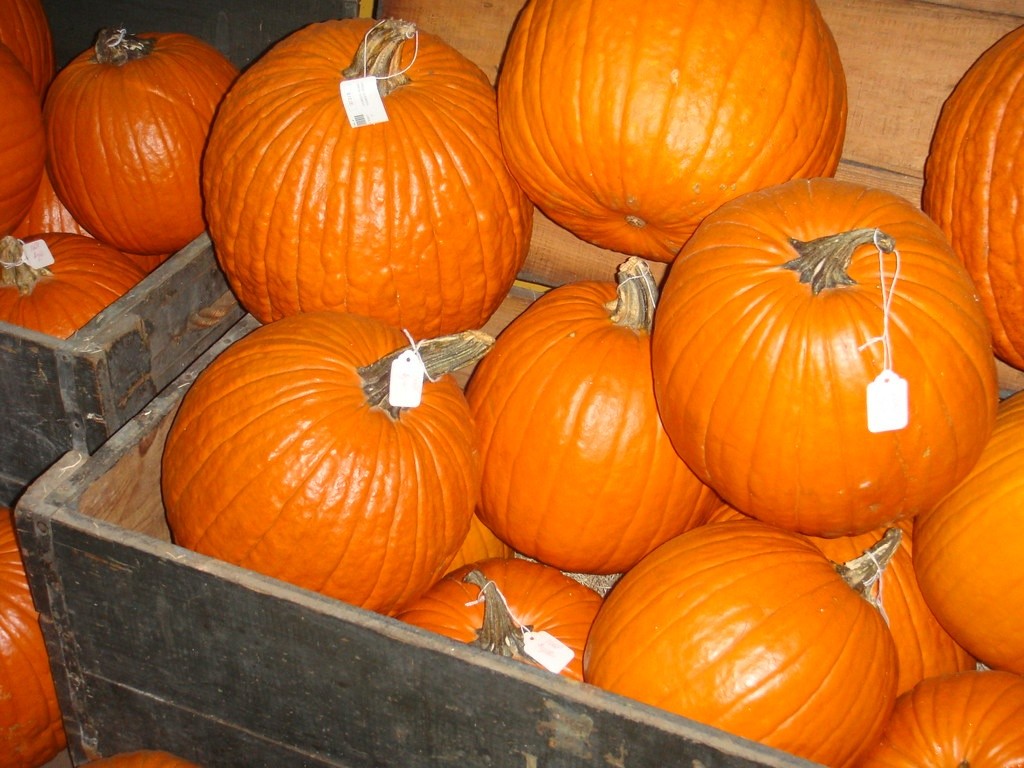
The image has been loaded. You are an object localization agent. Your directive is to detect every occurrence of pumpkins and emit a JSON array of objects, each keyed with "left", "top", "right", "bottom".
[{"left": 0, "top": 0, "right": 1024, "bottom": 768}]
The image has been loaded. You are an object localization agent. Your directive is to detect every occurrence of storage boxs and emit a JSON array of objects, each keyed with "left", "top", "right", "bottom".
[{"left": 0, "top": 0, "right": 1024, "bottom": 766}]
